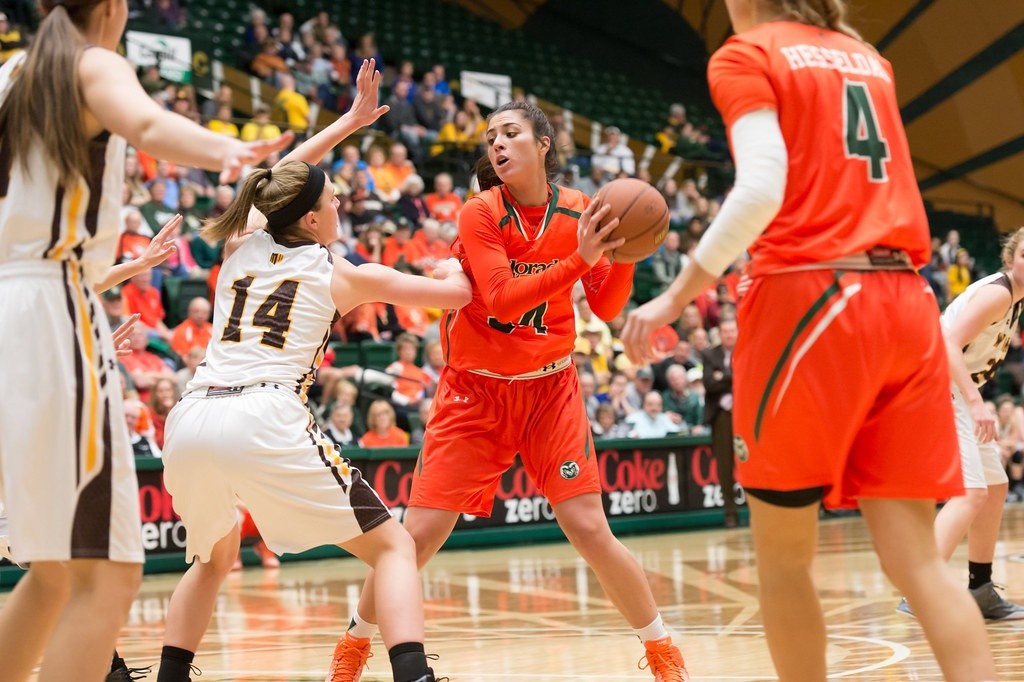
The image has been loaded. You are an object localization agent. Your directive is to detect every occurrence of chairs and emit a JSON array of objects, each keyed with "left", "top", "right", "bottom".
[{"left": 117, "top": 0, "right": 1024, "bottom": 443}]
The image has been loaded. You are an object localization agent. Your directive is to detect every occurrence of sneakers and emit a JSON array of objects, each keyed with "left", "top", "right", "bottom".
[
  {"left": 324, "top": 632, "right": 374, "bottom": 682},
  {"left": 638, "top": 636, "right": 689, "bottom": 682},
  {"left": 970, "top": 580, "right": 1024, "bottom": 619},
  {"left": 895, "top": 597, "right": 916, "bottom": 618}
]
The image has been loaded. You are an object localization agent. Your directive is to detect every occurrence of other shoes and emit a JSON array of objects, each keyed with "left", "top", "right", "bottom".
[
  {"left": 105, "top": 658, "right": 158, "bottom": 682},
  {"left": 408, "top": 667, "right": 449, "bottom": 682},
  {"left": 156, "top": 665, "right": 202, "bottom": 682}
]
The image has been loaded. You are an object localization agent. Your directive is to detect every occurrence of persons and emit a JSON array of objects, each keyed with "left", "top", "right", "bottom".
[
  {"left": 156, "top": 60, "right": 471, "bottom": 681},
  {"left": 0, "top": 0, "right": 294, "bottom": 681},
  {"left": 895, "top": 224, "right": 1024, "bottom": 620},
  {"left": 688, "top": 105, "right": 1023, "bottom": 486},
  {"left": 702, "top": 320, "right": 738, "bottom": 532},
  {"left": 323, "top": 101, "right": 688, "bottom": 682},
  {"left": 0, "top": 0, "right": 707, "bottom": 453},
  {"left": 621, "top": 1, "right": 997, "bottom": 681},
  {"left": 92, "top": 212, "right": 183, "bottom": 681}
]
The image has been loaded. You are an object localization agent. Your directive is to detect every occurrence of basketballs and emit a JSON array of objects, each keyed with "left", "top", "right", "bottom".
[{"left": 591, "top": 177, "right": 670, "bottom": 263}]
